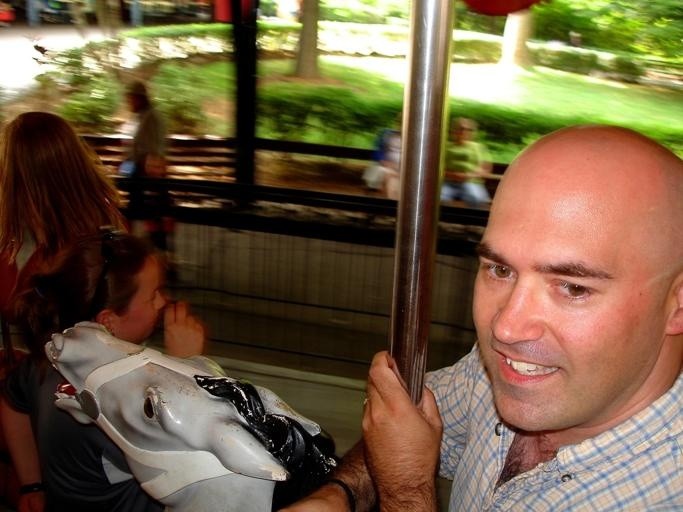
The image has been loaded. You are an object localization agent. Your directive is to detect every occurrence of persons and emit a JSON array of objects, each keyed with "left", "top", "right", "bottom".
[
  {"left": 439, "top": 112, "right": 491, "bottom": 205},
  {"left": 16, "top": 226, "right": 339, "bottom": 512},
  {"left": 362, "top": 129, "right": 400, "bottom": 201},
  {"left": 118, "top": 81, "right": 179, "bottom": 288},
  {"left": 0, "top": 111, "right": 131, "bottom": 512},
  {"left": 274, "top": 125, "right": 676, "bottom": 511}
]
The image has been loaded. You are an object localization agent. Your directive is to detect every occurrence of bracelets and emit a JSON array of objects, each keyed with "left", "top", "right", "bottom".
[{"left": 15, "top": 483, "right": 44, "bottom": 496}]
